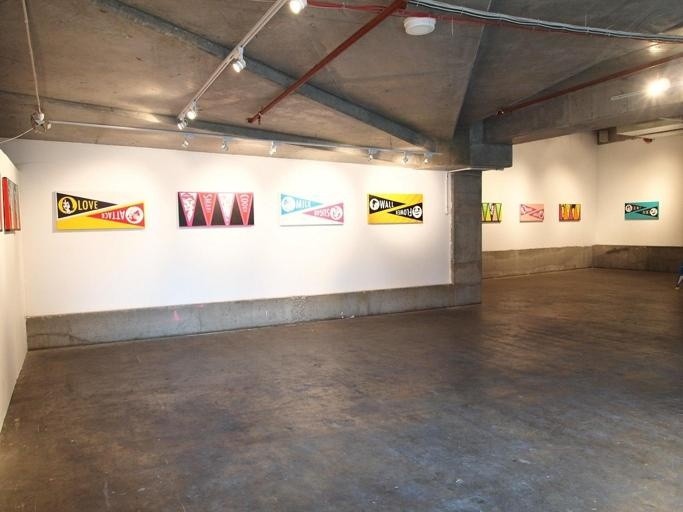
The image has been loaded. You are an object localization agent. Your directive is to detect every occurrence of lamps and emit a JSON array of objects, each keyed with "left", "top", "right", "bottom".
[
  {"left": 29, "top": 112, "right": 52, "bottom": 135},
  {"left": 178, "top": 132, "right": 280, "bottom": 158},
  {"left": 177, "top": 1, "right": 305, "bottom": 133},
  {"left": 364, "top": 146, "right": 433, "bottom": 167}
]
